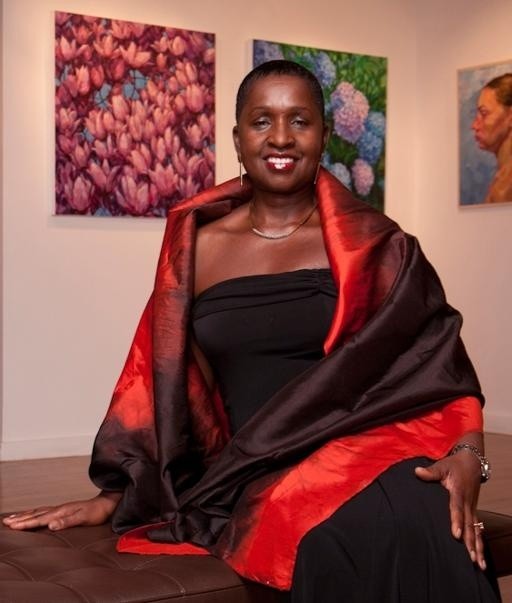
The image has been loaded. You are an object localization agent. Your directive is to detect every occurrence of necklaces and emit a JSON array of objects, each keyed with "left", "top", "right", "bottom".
[{"left": 249, "top": 197, "right": 318, "bottom": 239}]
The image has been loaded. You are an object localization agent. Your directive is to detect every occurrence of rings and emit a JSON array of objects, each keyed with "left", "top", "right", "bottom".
[{"left": 473, "top": 522, "right": 484, "bottom": 529}]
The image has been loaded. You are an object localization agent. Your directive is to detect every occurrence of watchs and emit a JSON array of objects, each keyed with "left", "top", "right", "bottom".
[{"left": 451, "top": 443, "right": 491, "bottom": 483}]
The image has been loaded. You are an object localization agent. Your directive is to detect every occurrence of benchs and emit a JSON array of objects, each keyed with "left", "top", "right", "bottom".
[{"left": 0, "top": 508, "right": 512, "bottom": 603}]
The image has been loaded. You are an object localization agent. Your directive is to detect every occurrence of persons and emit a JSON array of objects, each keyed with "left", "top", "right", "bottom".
[
  {"left": 0, "top": 60, "right": 512, "bottom": 602},
  {"left": 471, "top": 73, "right": 512, "bottom": 203}
]
